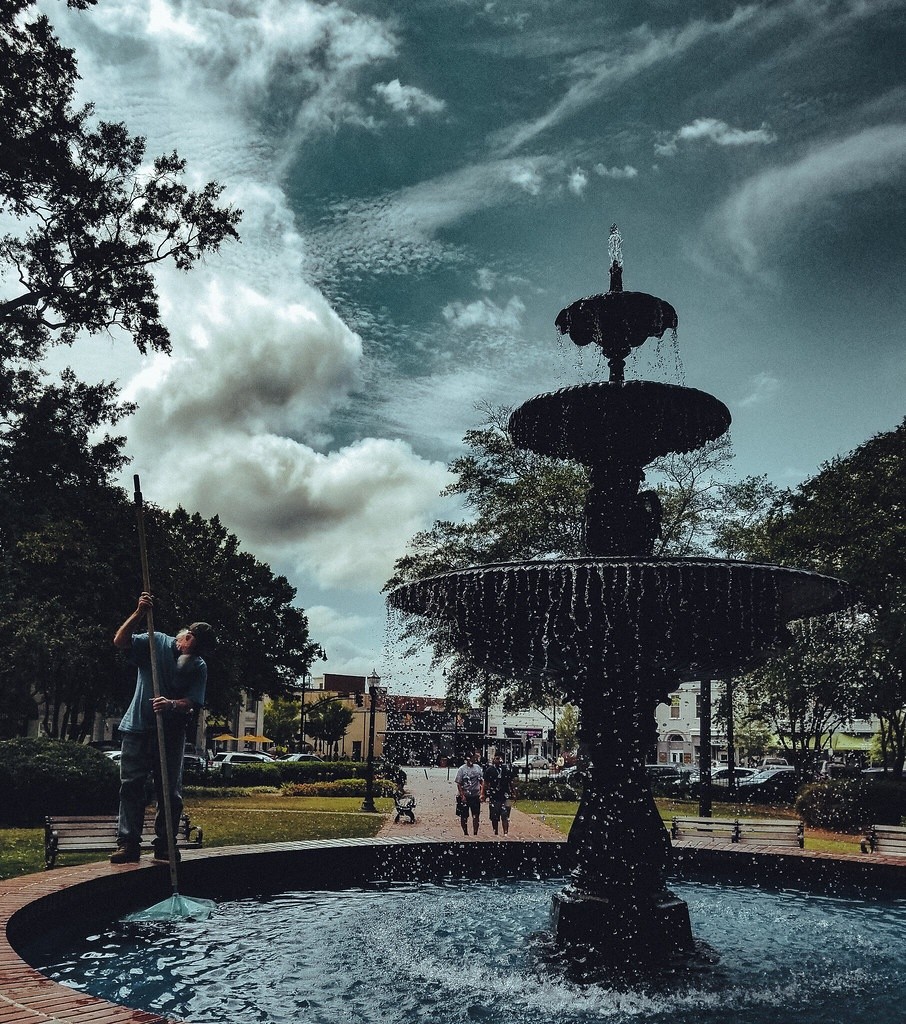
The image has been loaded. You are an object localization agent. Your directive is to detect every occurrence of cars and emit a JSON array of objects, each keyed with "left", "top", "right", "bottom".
[
  {"left": 697, "top": 758, "right": 793, "bottom": 786},
  {"left": 513, "top": 755, "right": 549, "bottom": 770},
  {"left": 103, "top": 743, "right": 325, "bottom": 768}
]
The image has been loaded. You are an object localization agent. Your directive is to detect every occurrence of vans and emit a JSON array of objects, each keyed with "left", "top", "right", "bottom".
[{"left": 643, "top": 765, "right": 679, "bottom": 783}]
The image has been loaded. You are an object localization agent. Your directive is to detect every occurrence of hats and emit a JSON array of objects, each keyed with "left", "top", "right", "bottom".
[{"left": 189, "top": 623, "right": 217, "bottom": 657}]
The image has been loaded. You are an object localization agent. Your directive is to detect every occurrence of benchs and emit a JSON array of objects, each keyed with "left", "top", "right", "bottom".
[
  {"left": 393, "top": 790, "right": 417, "bottom": 824},
  {"left": 669, "top": 815, "right": 807, "bottom": 849},
  {"left": 861, "top": 824, "right": 906, "bottom": 856},
  {"left": 44, "top": 816, "right": 204, "bottom": 871}
]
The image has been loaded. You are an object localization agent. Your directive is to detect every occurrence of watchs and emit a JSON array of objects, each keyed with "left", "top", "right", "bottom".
[{"left": 170, "top": 699, "right": 176, "bottom": 712}]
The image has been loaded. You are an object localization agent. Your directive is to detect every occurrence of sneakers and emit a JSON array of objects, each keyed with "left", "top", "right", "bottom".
[
  {"left": 154, "top": 843, "right": 181, "bottom": 861},
  {"left": 110, "top": 844, "right": 140, "bottom": 863}
]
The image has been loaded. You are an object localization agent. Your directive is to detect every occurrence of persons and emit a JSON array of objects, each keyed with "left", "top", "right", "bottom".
[
  {"left": 556, "top": 754, "right": 565, "bottom": 772},
  {"left": 108, "top": 591, "right": 214, "bottom": 864},
  {"left": 455, "top": 752, "right": 514, "bottom": 836},
  {"left": 334, "top": 750, "right": 357, "bottom": 762}
]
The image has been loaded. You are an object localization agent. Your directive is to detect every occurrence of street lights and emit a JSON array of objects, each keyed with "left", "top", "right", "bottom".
[
  {"left": 300, "top": 661, "right": 363, "bottom": 755},
  {"left": 360, "top": 668, "right": 381, "bottom": 812},
  {"left": 442, "top": 666, "right": 489, "bottom": 772}
]
